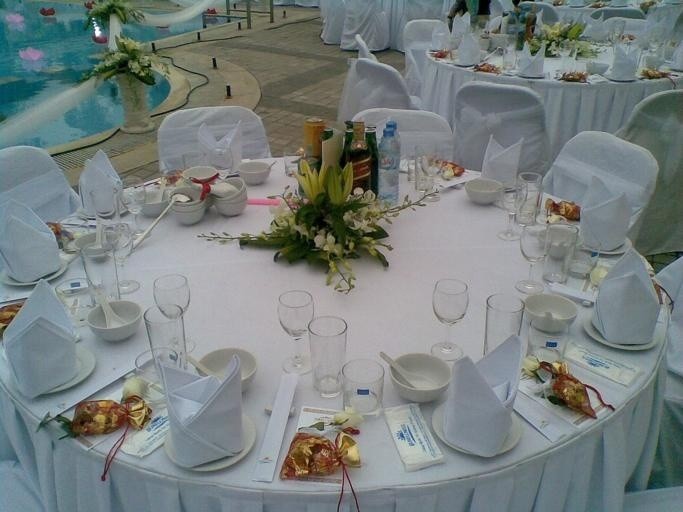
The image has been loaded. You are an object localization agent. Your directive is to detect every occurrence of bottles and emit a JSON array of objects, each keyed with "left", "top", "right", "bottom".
[
  {"left": 364, "top": 125, "right": 379, "bottom": 188},
  {"left": 321, "top": 129, "right": 333, "bottom": 141},
  {"left": 378, "top": 127, "right": 400, "bottom": 201},
  {"left": 348, "top": 122, "right": 371, "bottom": 192},
  {"left": 339, "top": 120, "right": 352, "bottom": 166},
  {"left": 380, "top": 121, "right": 401, "bottom": 160}
]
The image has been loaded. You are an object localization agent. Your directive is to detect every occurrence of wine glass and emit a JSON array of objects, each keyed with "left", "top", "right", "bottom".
[
  {"left": 514, "top": 224, "right": 552, "bottom": 295},
  {"left": 120, "top": 178, "right": 151, "bottom": 238},
  {"left": 103, "top": 223, "right": 139, "bottom": 295},
  {"left": 430, "top": 278, "right": 469, "bottom": 362},
  {"left": 420, "top": 153, "right": 441, "bottom": 200},
  {"left": 277, "top": 290, "right": 314, "bottom": 375},
  {"left": 496, "top": 180, "right": 528, "bottom": 241},
  {"left": 152, "top": 274, "right": 196, "bottom": 353},
  {"left": 214, "top": 147, "right": 233, "bottom": 180}
]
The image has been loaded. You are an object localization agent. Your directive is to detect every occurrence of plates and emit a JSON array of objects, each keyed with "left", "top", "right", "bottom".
[
  {"left": 40, "top": 344, "right": 96, "bottom": 394},
  {"left": 606, "top": 75, "right": 637, "bottom": 82},
  {"left": 453, "top": 62, "right": 474, "bottom": 67},
  {"left": 585, "top": 236, "right": 633, "bottom": 255},
  {"left": 583, "top": 316, "right": 657, "bottom": 350},
  {"left": 166, "top": 415, "right": 257, "bottom": 472},
  {"left": 518, "top": 72, "right": 546, "bottom": 79},
  {"left": 432, "top": 401, "right": 520, "bottom": 457},
  {"left": 0, "top": 259, "right": 68, "bottom": 286}
]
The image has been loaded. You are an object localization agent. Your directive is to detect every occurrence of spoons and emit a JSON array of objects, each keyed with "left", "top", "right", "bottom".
[
  {"left": 96, "top": 289, "right": 127, "bottom": 328},
  {"left": 153, "top": 178, "right": 166, "bottom": 202},
  {"left": 380, "top": 352, "right": 437, "bottom": 389}
]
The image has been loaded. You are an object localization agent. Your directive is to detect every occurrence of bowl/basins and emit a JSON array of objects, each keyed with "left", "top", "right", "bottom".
[
  {"left": 170, "top": 161, "right": 271, "bottom": 225},
  {"left": 142, "top": 190, "right": 169, "bottom": 217},
  {"left": 196, "top": 348, "right": 257, "bottom": 393},
  {"left": 524, "top": 293, "right": 578, "bottom": 333},
  {"left": 389, "top": 353, "right": 452, "bottom": 403},
  {"left": 465, "top": 178, "right": 502, "bottom": 205},
  {"left": 587, "top": 62, "right": 609, "bottom": 75},
  {"left": 87, "top": 300, "right": 142, "bottom": 341}
]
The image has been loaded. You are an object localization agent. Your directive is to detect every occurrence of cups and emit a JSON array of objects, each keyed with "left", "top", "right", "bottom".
[
  {"left": 183, "top": 151, "right": 205, "bottom": 170},
  {"left": 542, "top": 224, "right": 578, "bottom": 286},
  {"left": 527, "top": 317, "right": 570, "bottom": 361},
  {"left": 143, "top": 303, "right": 188, "bottom": 373},
  {"left": 135, "top": 347, "right": 182, "bottom": 407},
  {"left": 415, "top": 159, "right": 431, "bottom": 192},
  {"left": 565, "top": 241, "right": 602, "bottom": 278},
  {"left": 408, "top": 161, "right": 416, "bottom": 182},
  {"left": 428, "top": 8, "right": 676, "bottom": 86},
  {"left": 80, "top": 241, "right": 120, "bottom": 306},
  {"left": 515, "top": 172, "right": 542, "bottom": 224},
  {"left": 283, "top": 152, "right": 301, "bottom": 176},
  {"left": 55, "top": 278, "right": 96, "bottom": 326},
  {"left": 60, "top": 217, "right": 90, "bottom": 254},
  {"left": 341, "top": 358, "right": 384, "bottom": 419},
  {"left": 90, "top": 187, "right": 115, "bottom": 248},
  {"left": 309, "top": 316, "right": 347, "bottom": 397},
  {"left": 482, "top": 293, "right": 526, "bottom": 356}
]
